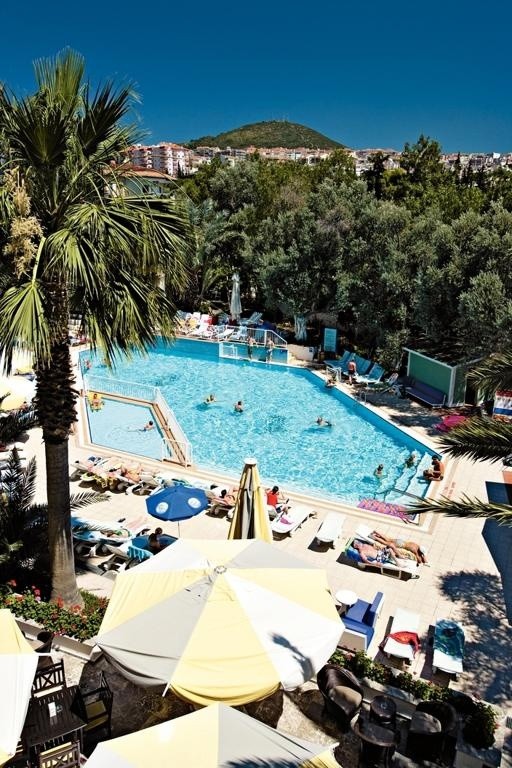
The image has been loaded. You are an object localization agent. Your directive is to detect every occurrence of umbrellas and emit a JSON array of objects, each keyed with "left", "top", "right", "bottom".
[
  {"left": 94, "top": 537, "right": 345, "bottom": 707},
  {"left": 230, "top": 272, "right": 242, "bottom": 315},
  {"left": 0, "top": 609, "right": 39, "bottom": 764},
  {"left": 78, "top": 706, "right": 342, "bottom": 768}
]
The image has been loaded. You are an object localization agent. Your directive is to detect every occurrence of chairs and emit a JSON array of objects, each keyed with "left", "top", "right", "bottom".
[
  {"left": 73, "top": 522, "right": 178, "bottom": 572},
  {"left": 70, "top": 457, "right": 242, "bottom": 519},
  {"left": 354, "top": 523, "right": 427, "bottom": 563},
  {"left": 270, "top": 503, "right": 316, "bottom": 538},
  {"left": 349, "top": 713, "right": 402, "bottom": 768},
  {"left": 173, "top": 306, "right": 275, "bottom": 340},
  {"left": 345, "top": 591, "right": 385, "bottom": 627},
  {"left": 323, "top": 350, "right": 447, "bottom": 408},
  {"left": 316, "top": 664, "right": 365, "bottom": 733},
  {"left": 337, "top": 617, "right": 375, "bottom": 654},
  {"left": 404, "top": 698, "right": 457, "bottom": 768},
  {"left": 314, "top": 511, "right": 347, "bottom": 550},
  {"left": 21, "top": 629, "right": 116, "bottom": 768},
  {"left": 344, "top": 537, "right": 418, "bottom": 580}
]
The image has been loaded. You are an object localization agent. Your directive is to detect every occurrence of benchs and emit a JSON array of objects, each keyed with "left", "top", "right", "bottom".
[
  {"left": 431, "top": 616, "right": 465, "bottom": 680},
  {"left": 379, "top": 609, "right": 421, "bottom": 672}
]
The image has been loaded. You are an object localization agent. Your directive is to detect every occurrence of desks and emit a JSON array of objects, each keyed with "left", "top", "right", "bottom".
[
  {"left": 334, "top": 589, "right": 358, "bottom": 617},
  {"left": 368, "top": 695, "right": 398, "bottom": 732}
]
